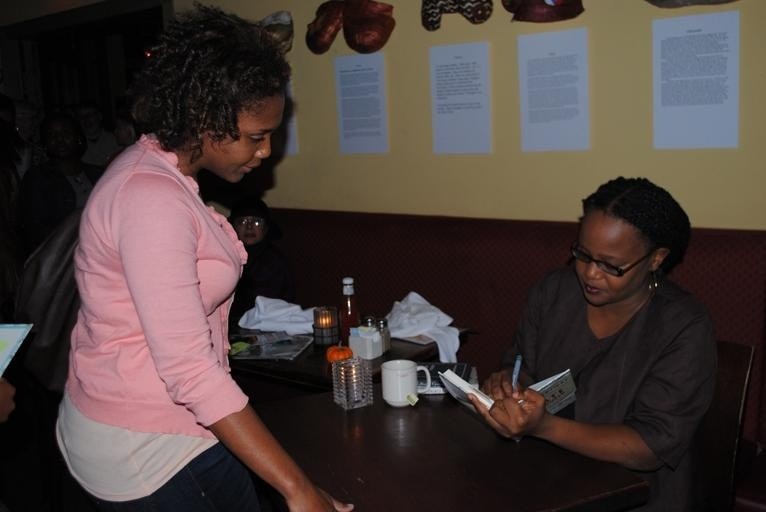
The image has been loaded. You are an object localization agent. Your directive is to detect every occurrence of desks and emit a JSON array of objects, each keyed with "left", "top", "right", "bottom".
[
  {"left": 242, "top": 386, "right": 652, "bottom": 512},
  {"left": 222, "top": 302, "right": 468, "bottom": 402}
]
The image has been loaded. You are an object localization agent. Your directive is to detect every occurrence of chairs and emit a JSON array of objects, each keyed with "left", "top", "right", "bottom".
[{"left": 671, "top": 336, "right": 758, "bottom": 512}]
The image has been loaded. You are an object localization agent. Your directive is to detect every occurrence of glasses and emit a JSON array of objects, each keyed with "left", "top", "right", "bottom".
[
  {"left": 232, "top": 218, "right": 268, "bottom": 231},
  {"left": 566, "top": 236, "right": 660, "bottom": 279}
]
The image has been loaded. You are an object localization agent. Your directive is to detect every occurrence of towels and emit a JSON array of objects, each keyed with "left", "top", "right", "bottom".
[
  {"left": 237, "top": 296, "right": 317, "bottom": 335},
  {"left": 381, "top": 290, "right": 460, "bottom": 365}
]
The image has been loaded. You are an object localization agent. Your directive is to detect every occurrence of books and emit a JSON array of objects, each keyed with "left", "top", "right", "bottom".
[{"left": 436, "top": 366, "right": 579, "bottom": 446}]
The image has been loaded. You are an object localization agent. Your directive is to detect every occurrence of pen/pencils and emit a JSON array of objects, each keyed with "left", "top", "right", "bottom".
[{"left": 511, "top": 354, "right": 522, "bottom": 388}]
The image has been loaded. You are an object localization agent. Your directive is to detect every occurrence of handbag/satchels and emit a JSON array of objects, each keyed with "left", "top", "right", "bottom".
[{"left": 12, "top": 205, "right": 86, "bottom": 394}]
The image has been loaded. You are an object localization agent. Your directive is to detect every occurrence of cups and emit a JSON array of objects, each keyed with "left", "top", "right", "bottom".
[
  {"left": 381, "top": 359, "right": 431, "bottom": 408},
  {"left": 235, "top": 216, "right": 264, "bottom": 246}
]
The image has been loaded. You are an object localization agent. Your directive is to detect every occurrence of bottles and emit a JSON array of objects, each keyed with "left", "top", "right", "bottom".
[
  {"left": 375, "top": 318, "right": 391, "bottom": 353},
  {"left": 362, "top": 315, "right": 376, "bottom": 328},
  {"left": 338, "top": 277, "right": 362, "bottom": 346}
]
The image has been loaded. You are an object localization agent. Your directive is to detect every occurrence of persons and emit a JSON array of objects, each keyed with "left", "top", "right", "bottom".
[
  {"left": 1, "top": 92, "right": 138, "bottom": 313},
  {"left": 466, "top": 176, "right": 719, "bottom": 512},
  {"left": 56, "top": 5, "right": 356, "bottom": 512},
  {"left": 0, "top": 376, "right": 20, "bottom": 420},
  {"left": 225, "top": 196, "right": 293, "bottom": 336}
]
